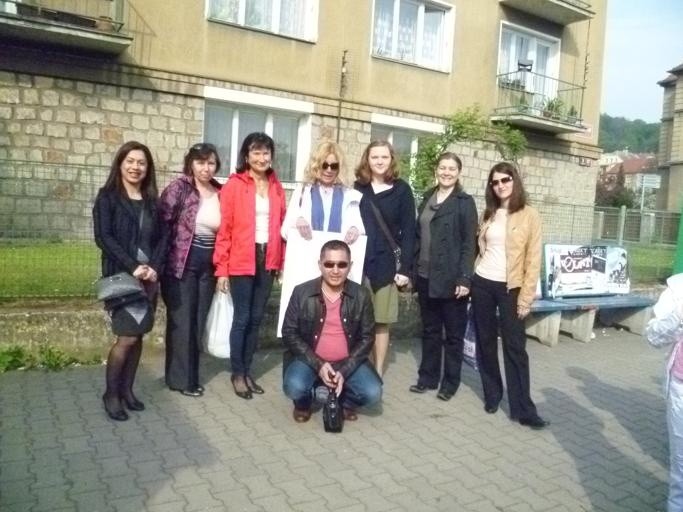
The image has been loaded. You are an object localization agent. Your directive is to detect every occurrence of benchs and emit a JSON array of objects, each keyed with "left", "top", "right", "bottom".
[{"left": 530, "top": 296, "right": 656, "bottom": 347}]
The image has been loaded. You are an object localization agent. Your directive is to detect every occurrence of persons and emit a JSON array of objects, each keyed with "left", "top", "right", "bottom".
[
  {"left": 470, "top": 162, "right": 548, "bottom": 430},
  {"left": 352, "top": 140, "right": 416, "bottom": 382},
  {"left": 282, "top": 241, "right": 383, "bottom": 422},
  {"left": 280, "top": 142, "right": 366, "bottom": 246},
  {"left": 211, "top": 132, "right": 287, "bottom": 401},
  {"left": 647, "top": 270, "right": 678, "bottom": 508},
  {"left": 90, "top": 140, "right": 166, "bottom": 422},
  {"left": 409, "top": 153, "right": 478, "bottom": 401},
  {"left": 160, "top": 143, "right": 224, "bottom": 398}
]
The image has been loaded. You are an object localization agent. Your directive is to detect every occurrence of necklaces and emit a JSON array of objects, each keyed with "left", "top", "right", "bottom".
[{"left": 322, "top": 186, "right": 332, "bottom": 194}]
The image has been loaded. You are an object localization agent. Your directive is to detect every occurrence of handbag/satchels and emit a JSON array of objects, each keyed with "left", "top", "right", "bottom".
[
  {"left": 94, "top": 199, "right": 153, "bottom": 310},
  {"left": 362, "top": 187, "right": 414, "bottom": 292},
  {"left": 202, "top": 281, "right": 234, "bottom": 360},
  {"left": 322, "top": 376, "right": 343, "bottom": 432}
]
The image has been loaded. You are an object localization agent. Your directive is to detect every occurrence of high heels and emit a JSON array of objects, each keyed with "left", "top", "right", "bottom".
[
  {"left": 119, "top": 389, "right": 144, "bottom": 410},
  {"left": 244, "top": 374, "right": 263, "bottom": 394},
  {"left": 102, "top": 392, "right": 128, "bottom": 420},
  {"left": 230, "top": 374, "right": 252, "bottom": 398}
]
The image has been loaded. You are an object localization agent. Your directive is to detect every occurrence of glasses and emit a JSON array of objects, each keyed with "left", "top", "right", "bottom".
[
  {"left": 489, "top": 175, "right": 512, "bottom": 185},
  {"left": 193, "top": 143, "right": 216, "bottom": 151},
  {"left": 321, "top": 161, "right": 339, "bottom": 170},
  {"left": 322, "top": 261, "right": 349, "bottom": 268}
]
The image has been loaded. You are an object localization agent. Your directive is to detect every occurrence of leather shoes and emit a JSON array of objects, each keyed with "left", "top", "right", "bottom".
[
  {"left": 190, "top": 383, "right": 203, "bottom": 392},
  {"left": 519, "top": 415, "right": 550, "bottom": 427},
  {"left": 410, "top": 381, "right": 438, "bottom": 393},
  {"left": 341, "top": 407, "right": 357, "bottom": 420},
  {"left": 436, "top": 388, "right": 455, "bottom": 401},
  {"left": 169, "top": 385, "right": 202, "bottom": 395},
  {"left": 484, "top": 394, "right": 502, "bottom": 413},
  {"left": 293, "top": 406, "right": 311, "bottom": 422}
]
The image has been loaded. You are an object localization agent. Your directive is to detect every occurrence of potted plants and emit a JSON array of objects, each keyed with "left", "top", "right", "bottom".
[
  {"left": 543, "top": 98, "right": 562, "bottom": 119},
  {"left": 566, "top": 107, "right": 577, "bottom": 124},
  {"left": 518, "top": 93, "right": 528, "bottom": 113}
]
